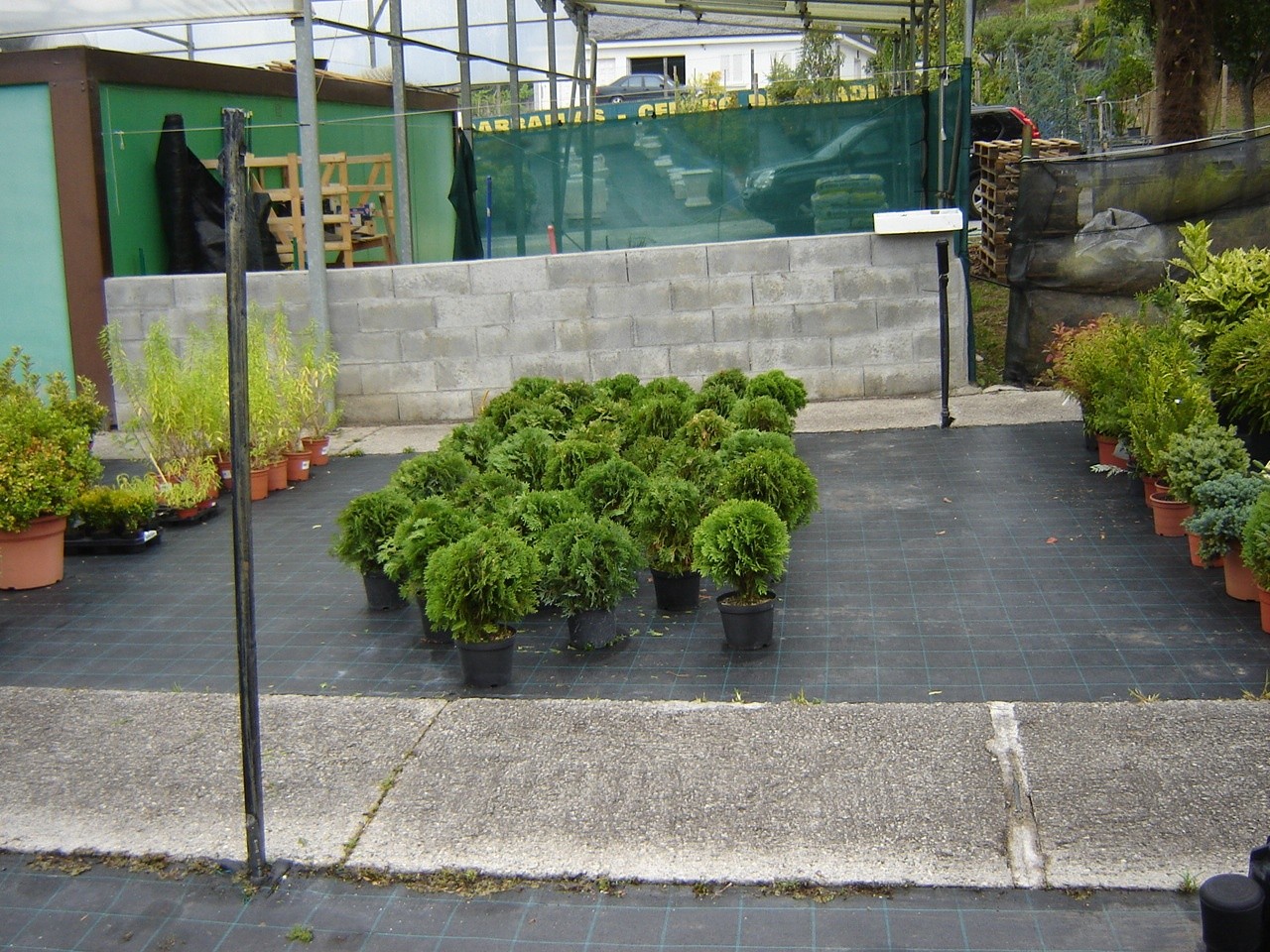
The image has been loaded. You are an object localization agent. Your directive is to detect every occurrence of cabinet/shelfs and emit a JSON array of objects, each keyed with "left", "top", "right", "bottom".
[{"left": 197, "top": 152, "right": 396, "bottom": 270}]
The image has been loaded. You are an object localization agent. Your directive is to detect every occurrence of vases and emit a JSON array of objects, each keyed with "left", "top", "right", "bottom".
[{"left": 290, "top": 59, "right": 330, "bottom": 70}]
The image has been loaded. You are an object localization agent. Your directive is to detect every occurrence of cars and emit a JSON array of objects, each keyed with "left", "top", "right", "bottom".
[{"left": 596, "top": 73, "right": 707, "bottom": 105}]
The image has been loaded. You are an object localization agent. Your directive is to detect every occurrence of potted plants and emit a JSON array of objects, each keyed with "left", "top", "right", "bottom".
[
  {"left": 0, "top": 296, "right": 822, "bottom": 691},
  {"left": 1116, "top": 56, "right": 1155, "bottom": 138},
  {"left": 1024, "top": 219, "right": 1270, "bottom": 633},
  {"left": 476, "top": 161, "right": 536, "bottom": 230}
]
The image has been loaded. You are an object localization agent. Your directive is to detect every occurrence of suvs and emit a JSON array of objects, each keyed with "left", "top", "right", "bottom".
[{"left": 742, "top": 105, "right": 1041, "bottom": 235}]
